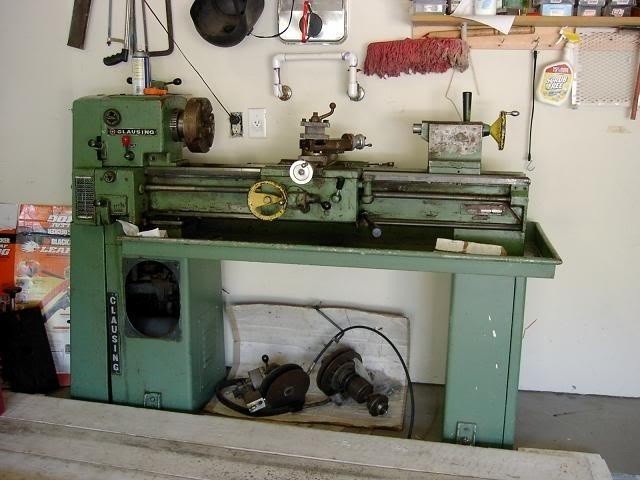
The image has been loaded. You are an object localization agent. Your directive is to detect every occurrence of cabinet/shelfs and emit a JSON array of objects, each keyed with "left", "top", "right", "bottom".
[{"left": 410, "top": 10, "right": 640, "bottom": 49}]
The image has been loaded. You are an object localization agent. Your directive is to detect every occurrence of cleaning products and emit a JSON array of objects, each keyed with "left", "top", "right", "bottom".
[{"left": 535, "top": 27, "right": 583, "bottom": 109}]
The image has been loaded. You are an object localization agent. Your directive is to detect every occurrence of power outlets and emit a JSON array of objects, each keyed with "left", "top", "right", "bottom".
[
  {"left": 247, "top": 108, "right": 267, "bottom": 139},
  {"left": 229, "top": 112, "right": 244, "bottom": 138}
]
